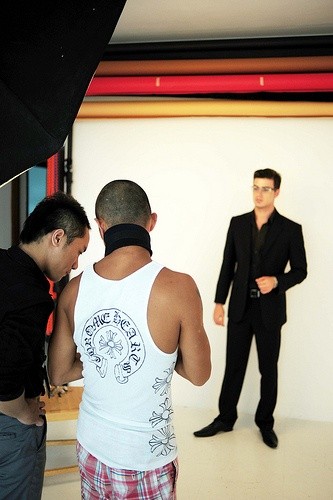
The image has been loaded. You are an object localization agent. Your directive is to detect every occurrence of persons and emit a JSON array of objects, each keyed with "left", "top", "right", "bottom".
[
  {"left": 48, "top": 178, "right": 212, "bottom": 500},
  {"left": 193, "top": 167, "right": 307, "bottom": 449},
  {"left": 0, "top": 194, "right": 90, "bottom": 500},
  {"left": 44, "top": 273, "right": 70, "bottom": 394}
]
